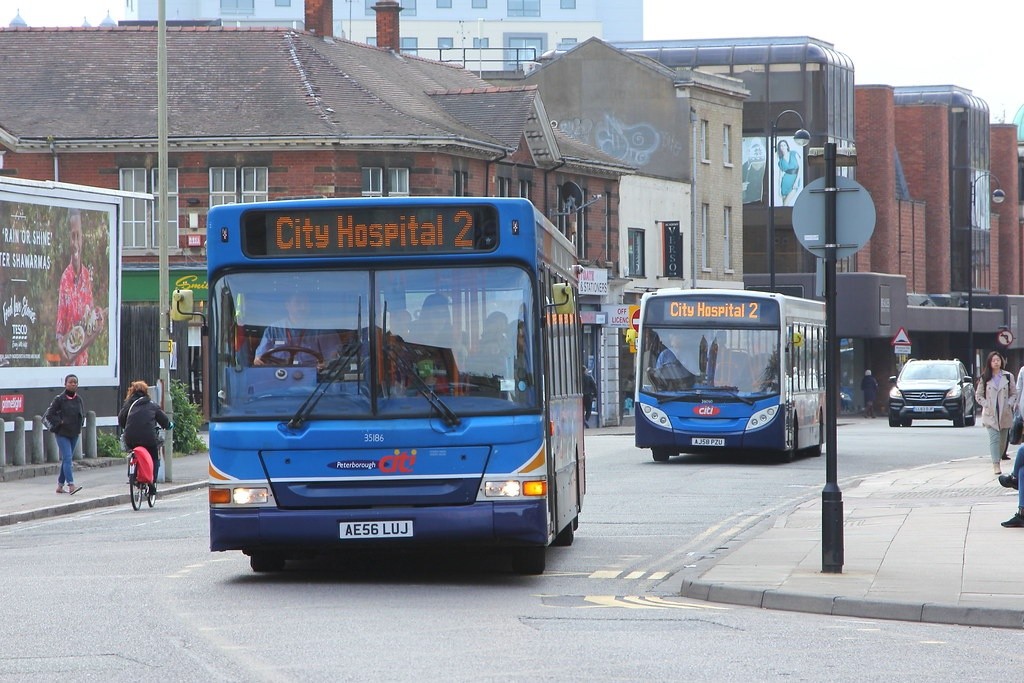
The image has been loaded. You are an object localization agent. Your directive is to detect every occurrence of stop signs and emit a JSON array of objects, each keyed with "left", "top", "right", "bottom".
[{"left": 632, "top": 309, "right": 640, "bottom": 332}]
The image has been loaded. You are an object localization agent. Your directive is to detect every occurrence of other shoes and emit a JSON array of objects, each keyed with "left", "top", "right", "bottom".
[
  {"left": 993, "top": 463, "right": 1001, "bottom": 474},
  {"left": 149, "top": 486, "right": 157, "bottom": 495},
  {"left": 1002, "top": 456, "right": 1010, "bottom": 460},
  {"left": 585, "top": 421, "right": 589, "bottom": 428}
]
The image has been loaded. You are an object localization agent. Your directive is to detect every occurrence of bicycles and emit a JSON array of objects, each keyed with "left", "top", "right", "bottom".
[{"left": 128, "top": 427, "right": 173, "bottom": 511}]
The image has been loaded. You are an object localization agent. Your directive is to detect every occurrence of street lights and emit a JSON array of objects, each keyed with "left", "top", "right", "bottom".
[
  {"left": 968, "top": 173, "right": 1006, "bottom": 374},
  {"left": 770, "top": 110, "right": 811, "bottom": 292}
]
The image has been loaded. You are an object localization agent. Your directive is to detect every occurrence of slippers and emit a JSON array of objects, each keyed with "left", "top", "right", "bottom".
[
  {"left": 69, "top": 486, "right": 82, "bottom": 495},
  {"left": 55, "top": 488, "right": 69, "bottom": 493}
]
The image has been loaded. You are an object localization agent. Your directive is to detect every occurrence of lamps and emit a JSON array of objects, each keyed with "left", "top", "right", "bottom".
[{"left": 186, "top": 198, "right": 200, "bottom": 204}]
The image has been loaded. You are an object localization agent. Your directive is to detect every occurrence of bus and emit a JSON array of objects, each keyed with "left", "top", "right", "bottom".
[
  {"left": 170, "top": 196, "right": 586, "bottom": 572},
  {"left": 634, "top": 289, "right": 824, "bottom": 463}
]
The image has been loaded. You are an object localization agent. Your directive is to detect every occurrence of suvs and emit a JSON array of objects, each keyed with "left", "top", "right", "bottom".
[{"left": 888, "top": 358, "right": 975, "bottom": 427}]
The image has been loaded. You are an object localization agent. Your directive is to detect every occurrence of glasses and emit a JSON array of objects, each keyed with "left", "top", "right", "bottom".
[{"left": 426, "top": 309, "right": 450, "bottom": 320}]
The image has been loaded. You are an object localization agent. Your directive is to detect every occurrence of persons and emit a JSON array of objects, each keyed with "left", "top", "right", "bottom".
[
  {"left": 117, "top": 381, "right": 173, "bottom": 495},
  {"left": 975, "top": 351, "right": 1017, "bottom": 474},
  {"left": 253, "top": 288, "right": 350, "bottom": 376},
  {"left": 382, "top": 294, "right": 535, "bottom": 404},
  {"left": 777, "top": 140, "right": 802, "bottom": 206},
  {"left": 999, "top": 367, "right": 1024, "bottom": 528},
  {"left": 582, "top": 365, "right": 598, "bottom": 427},
  {"left": 55, "top": 207, "right": 104, "bottom": 365},
  {"left": 861, "top": 370, "right": 878, "bottom": 418},
  {"left": 624, "top": 376, "right": 635, "bottom": 408},
  {"left": 739, "top": 138, "right": 766, "bottom": 202},
  {"left": 653, "top": 334, "right": 692, "bottom": 390},
  {"left": 46, "top": 375, "right": 85, "bottom": 494}
]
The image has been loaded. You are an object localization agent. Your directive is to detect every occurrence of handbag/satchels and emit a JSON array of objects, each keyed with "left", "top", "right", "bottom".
[
  {"left": 1009, "top": 414, "right": 1024, "bottom": 444},
  {"left": 120, "top": 433, "right": 130, "bottom": 451},
  {"left": 40, "top": 394, "right": 63, "bottom": 433}
]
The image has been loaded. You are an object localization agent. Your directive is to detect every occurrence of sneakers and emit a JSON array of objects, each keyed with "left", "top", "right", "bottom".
[
  {"left": 998, "top": 476, "right": 1019, "bottom": 490},
  {"left": 1001, "top": 512, "right": 1024, "bottom": 528}
]
